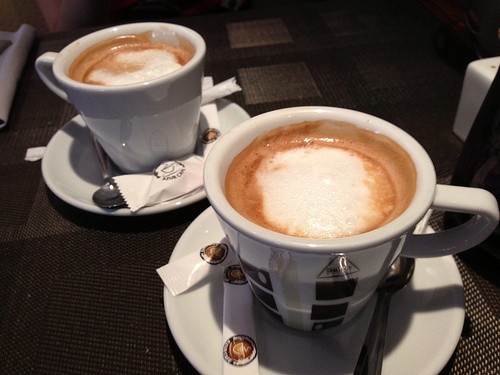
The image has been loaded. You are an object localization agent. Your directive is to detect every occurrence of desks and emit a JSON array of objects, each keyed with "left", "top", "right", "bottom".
[{"left": 0, "top": 0, "right": 500, "bottom": 375}]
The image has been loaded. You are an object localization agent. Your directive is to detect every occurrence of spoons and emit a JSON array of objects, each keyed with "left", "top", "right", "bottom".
[
  {"left": 92, "top": 138, "right": 127, "bottom": 208},
  {"left": 353, "top": 257, "right": 416, "bottom": 375}
]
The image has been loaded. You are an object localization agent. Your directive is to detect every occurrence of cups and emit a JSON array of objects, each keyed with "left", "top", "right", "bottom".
[
  {"left": 35, "top": 22, "right": 207, "bottom": 173},
  {"left": 204, "top": 106, "right": 499, "bottom": 332}
]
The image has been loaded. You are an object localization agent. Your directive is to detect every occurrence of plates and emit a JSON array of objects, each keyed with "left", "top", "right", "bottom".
[
  {"left": 41, "top": 99, "right": 252, "bottom": 216},
  {"left": 164, "top": 206, "right": 464, "bottom": 375}
]
